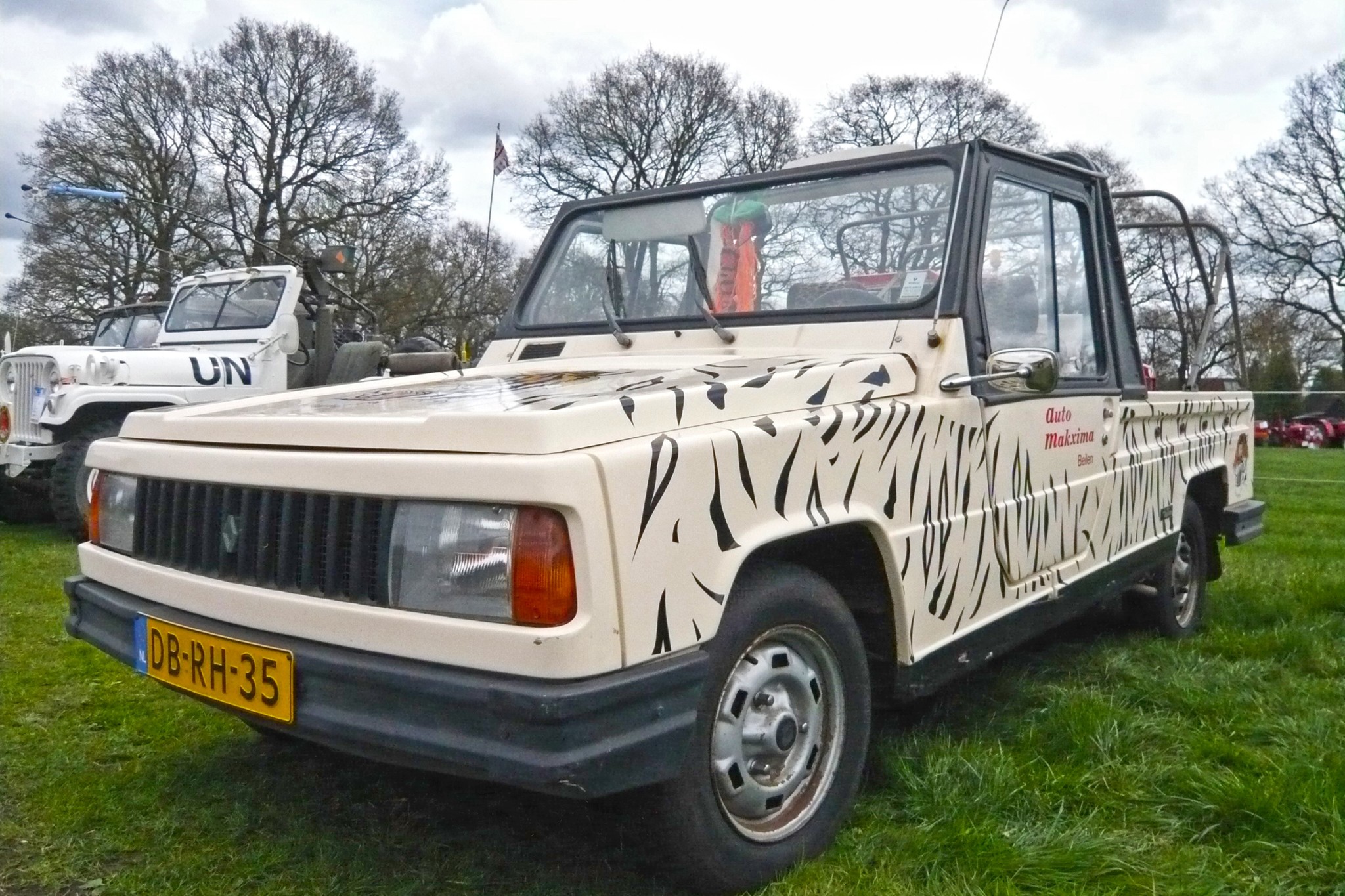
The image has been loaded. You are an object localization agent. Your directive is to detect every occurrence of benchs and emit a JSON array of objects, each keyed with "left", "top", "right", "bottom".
[
  {"left": 788, "top": 267, "right": 1041, "bottom": 357},
  {"left": 383, "top": 351, "right": 459, "bottom": 373}
]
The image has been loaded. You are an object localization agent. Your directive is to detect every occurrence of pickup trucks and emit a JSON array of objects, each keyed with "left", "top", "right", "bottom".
[{"left": 2, "top": 132, "right": 1268, "bottom": 895}]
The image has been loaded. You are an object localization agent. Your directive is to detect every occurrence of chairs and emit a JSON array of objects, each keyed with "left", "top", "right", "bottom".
[
  {"left": 285, "top": 348, "right": 315, "bottom": 387},
  {"left": 325, "top": 338, "right": 384, "bottom": 387}
]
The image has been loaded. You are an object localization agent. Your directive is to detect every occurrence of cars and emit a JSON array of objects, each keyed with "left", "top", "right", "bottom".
[{"left": 1255, "top": 411, "right": 1345, "bottom": 450}]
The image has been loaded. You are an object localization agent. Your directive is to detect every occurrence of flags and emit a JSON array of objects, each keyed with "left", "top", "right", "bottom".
[{"left": 492, "top": 134, "right": 511, "bottom": 176}]
